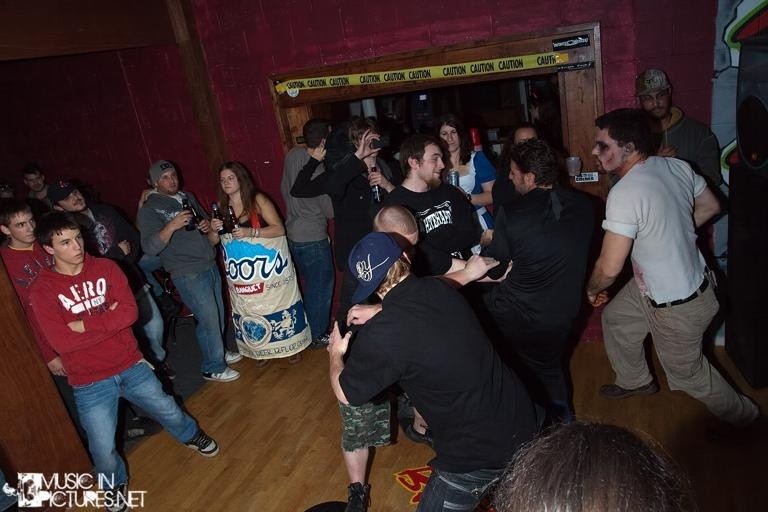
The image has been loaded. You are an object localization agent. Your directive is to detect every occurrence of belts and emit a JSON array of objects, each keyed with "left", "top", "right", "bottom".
[{"left": 648, "top": 266, "right": 708, "bottom": 308}]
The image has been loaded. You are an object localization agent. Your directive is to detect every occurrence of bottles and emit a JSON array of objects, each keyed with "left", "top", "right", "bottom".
[
  {"left": 371, "top": 164, "right": 385, "bottom": 206},
  {"left": 182, "top": 198, "right": 196, "bottom": 231},
  {"left": 211, "top": 201, "right": 240, "bottom": 235}
]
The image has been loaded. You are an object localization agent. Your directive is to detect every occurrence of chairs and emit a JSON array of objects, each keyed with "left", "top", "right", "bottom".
[{"left": 161, "top": 273, "right": 198, "bottom": 345}]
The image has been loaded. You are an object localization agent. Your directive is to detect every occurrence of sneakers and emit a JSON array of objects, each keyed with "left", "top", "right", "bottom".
[
  {"left": 312, "top": 332, "right": 329, "bottom": 346},
  {"left": 104, "top": 483, "right": 128, "bottom": 512},
  {"left": 183, "top": 432, "right": 219, "bottom": 458},
  {"left": 201, "top": 365, "right": 240, "bottom": 383},
  {"left": 126, "top": 416, "right": 156, "bottom": 438},
  {"left": 344, "top": 482, "right": 371, "bottom": 512},
  {"left": 399, "top": 416, "right": 434, "bottom": 450},
  {"left": 223, "top": 350, "right": 243, "bottom": 365},
  {"left": 158, "top": 362, "right": 176, "bottom": 378}
]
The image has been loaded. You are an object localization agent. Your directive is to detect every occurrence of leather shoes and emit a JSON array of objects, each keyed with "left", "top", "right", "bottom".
[{"left": 600, "top": 379, "right": 658, "bottom": 400}]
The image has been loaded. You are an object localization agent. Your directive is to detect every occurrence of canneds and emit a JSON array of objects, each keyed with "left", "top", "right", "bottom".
[{"left": 448, "top": 171, "right": 458, "bottom": 187}]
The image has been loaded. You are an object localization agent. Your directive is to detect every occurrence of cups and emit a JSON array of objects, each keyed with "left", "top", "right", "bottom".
[{"left": 567, "top": 157, "right": 581, "bottom": 176}]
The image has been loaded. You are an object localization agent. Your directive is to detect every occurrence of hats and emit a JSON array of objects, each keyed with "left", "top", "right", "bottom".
[
  {"left": 632, "top": 68, "right": 671, "bottom": 97},
  {"left": 47, "top": 178, "right": 76, "bottom": 203},
  {"left": 148, "top": 159, "right": 175, "bottom": 185},
  {"left": 347, "top": 231, "right": 403, "bottom": 304}
]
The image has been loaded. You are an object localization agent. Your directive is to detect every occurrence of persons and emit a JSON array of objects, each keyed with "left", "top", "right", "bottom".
[{"left": 0, "top": 67, "right": 760, "bottom": 512}]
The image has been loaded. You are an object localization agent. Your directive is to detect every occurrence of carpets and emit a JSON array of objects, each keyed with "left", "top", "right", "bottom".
[{"left": 120, "top": 316, "right": 239, "bottom": 459}]
